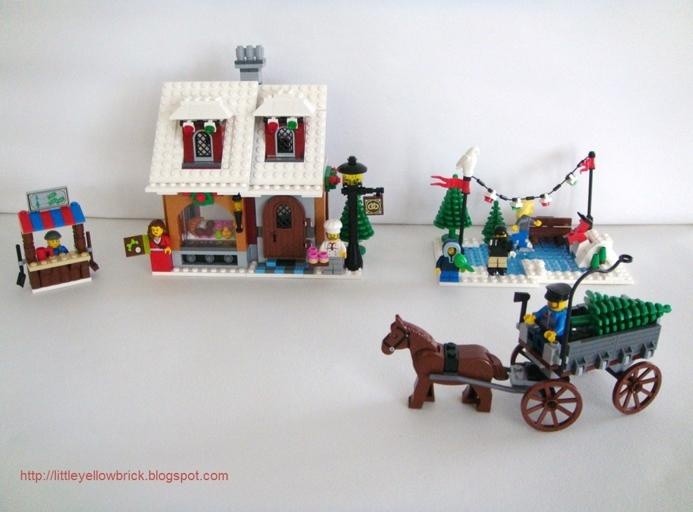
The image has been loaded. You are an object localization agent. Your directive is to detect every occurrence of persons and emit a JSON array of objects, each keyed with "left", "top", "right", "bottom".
[{"left": 13, "top": 184, "right": 99, "bottom": 296}]
